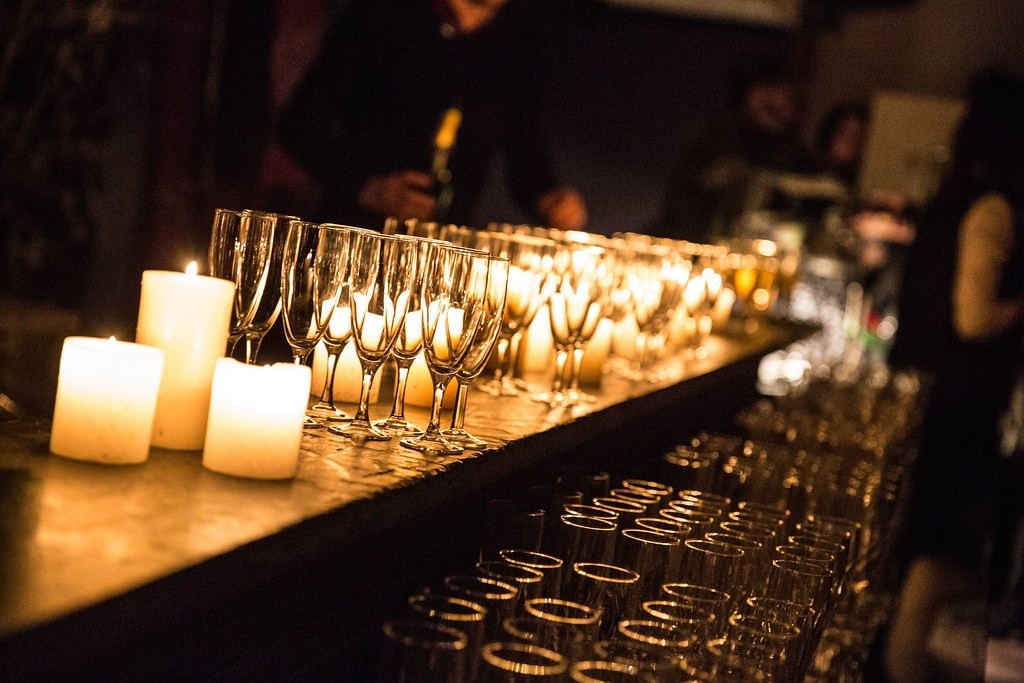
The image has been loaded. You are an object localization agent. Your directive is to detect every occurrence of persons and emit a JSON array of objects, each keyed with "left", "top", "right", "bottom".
[
  {"left": 653, "top": 56, "right": 1024, "bottom": 683},
  {"left": 278, "top": 0, "right": 589, "bottom": 237}
]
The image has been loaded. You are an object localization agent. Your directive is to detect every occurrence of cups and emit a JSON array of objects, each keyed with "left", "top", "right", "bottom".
[{"left": 366, "top": 373, "right": 930, "bottom": 683}]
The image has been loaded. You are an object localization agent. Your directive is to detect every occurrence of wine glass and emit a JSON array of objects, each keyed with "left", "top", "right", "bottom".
[{"left": 205, "top": 206, "right": 804, "bottom": 456}]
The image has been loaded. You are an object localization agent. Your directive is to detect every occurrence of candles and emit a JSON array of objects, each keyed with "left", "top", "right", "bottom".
[{"left": 48, "top": 257, "right": 314, "bottom": 480}]
